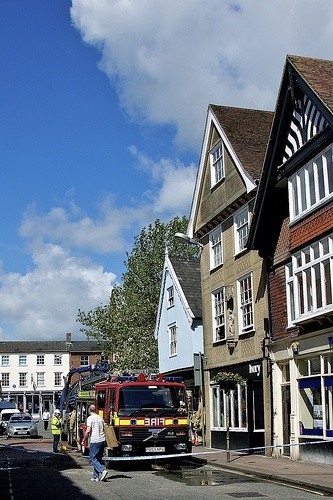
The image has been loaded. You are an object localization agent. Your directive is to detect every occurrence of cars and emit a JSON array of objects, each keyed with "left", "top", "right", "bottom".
[
  {"left": 6, "top": 413, "right": 38, "bottom": 439},
  {"left": 0, "top": 409, "right": 21, "bottom": 436}
]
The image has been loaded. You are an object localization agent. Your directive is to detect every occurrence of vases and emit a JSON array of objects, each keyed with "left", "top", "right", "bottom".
[{"left": 218, "top": 380, "right": 238, "bottom": 390}]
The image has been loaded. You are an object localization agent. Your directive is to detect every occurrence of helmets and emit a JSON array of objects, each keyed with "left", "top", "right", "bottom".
[{"left": 54, "top": 409, "right": 60, "bottom": 414}]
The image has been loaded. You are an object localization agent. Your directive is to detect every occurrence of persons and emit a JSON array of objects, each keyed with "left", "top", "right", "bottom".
[
  {"left": 43, "top": 409, "right": 51, "bottom": 430},
  {"left": 81, "top": 405, "right": 108, "bottom": 481},
  {"left": 51, "top": 409, "right": 62, "bottom": 452}
]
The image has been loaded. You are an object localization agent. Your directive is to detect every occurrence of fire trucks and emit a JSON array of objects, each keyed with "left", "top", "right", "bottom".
[{"left": 57, "top": 360, "right": 201, "bottom": 468}]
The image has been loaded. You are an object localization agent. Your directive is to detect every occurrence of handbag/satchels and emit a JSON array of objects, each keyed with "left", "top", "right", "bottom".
[{"left": 104, "top": 424, "right": 118, "bottom": 447}]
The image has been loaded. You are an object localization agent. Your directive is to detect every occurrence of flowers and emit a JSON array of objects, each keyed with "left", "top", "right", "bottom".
[{"left": 213, "top": 372, "right": 245, "bottom": 384}]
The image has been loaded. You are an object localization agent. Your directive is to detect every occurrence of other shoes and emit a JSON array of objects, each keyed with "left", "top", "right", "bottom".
[
  {"left": 91, "top": 477, "right": 99, "bottom": 482},
  {"left": 54, "top": 449, "right": 60, "bottom": 453},
  {"left": 100, "top": 470, "right": 108, "bottom": 481}
]
